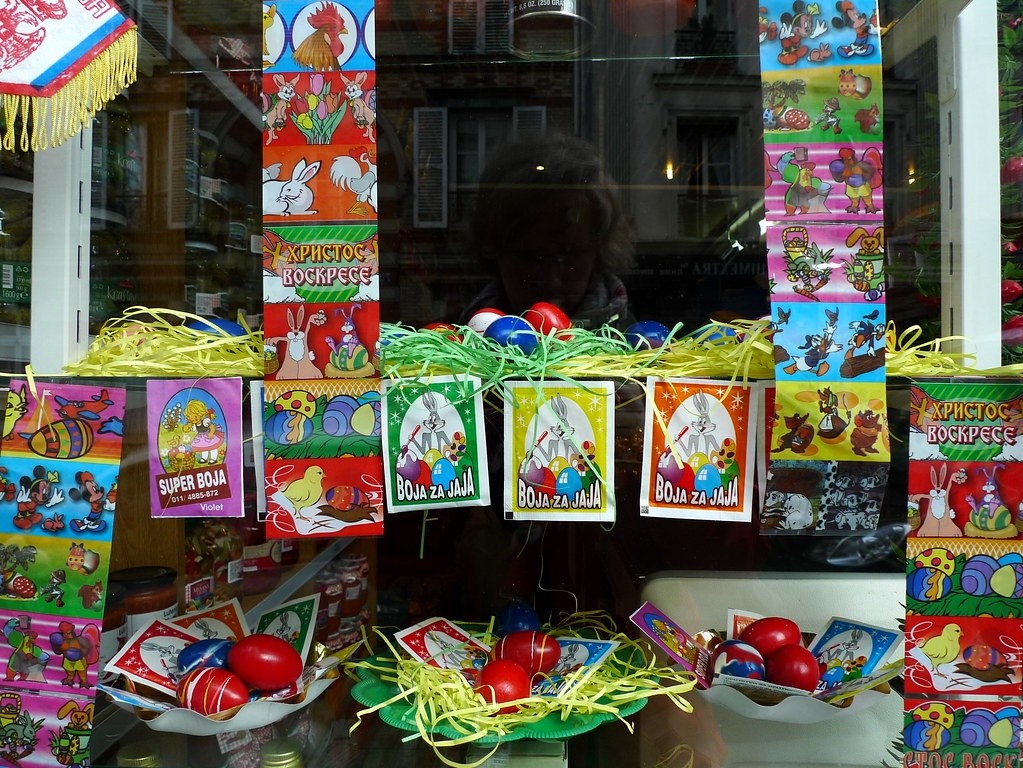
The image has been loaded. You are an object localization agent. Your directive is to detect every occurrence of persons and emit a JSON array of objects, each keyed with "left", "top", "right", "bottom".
[{"left": 456, "top": 134, "right": 641, "bottom": 331}]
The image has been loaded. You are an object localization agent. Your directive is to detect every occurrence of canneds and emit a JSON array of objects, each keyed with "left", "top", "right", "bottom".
[
  {"left": 1, "top": 84, "right": 263, "bottom": 334},
  {"left": 94, "top": 565, "right": 180, "bottom": 699}
]
